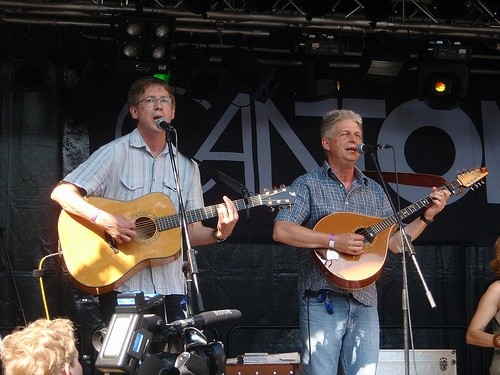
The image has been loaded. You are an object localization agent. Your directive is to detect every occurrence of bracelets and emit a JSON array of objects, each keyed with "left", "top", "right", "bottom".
[
  {"left": 493, "top": 333, "right": 500, "bottom": 348},
  {"left": 420, "top": 214, "right": 431, "bottom": 225},
  {"left": 91, "top": 208, "right": 100, "bottom": 223},
  {"left": 328, "top": 233, "right": 335, "bottom": 248},
  {"left": 212, "top": 229, "right": 226, "bottom": 242}
]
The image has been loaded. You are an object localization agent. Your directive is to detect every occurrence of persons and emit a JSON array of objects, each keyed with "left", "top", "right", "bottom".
[
  {"left": 466, "top": 236, "right": 500, "bottom": 375},
  {"left": 51, "top": 76, "right": 239, "bottom": 355},
  {"left": 273, "top": 110, "right": 451, "bottom": 375},
  {"left": 0, "top": 318, "right": 82, "bottom": 375}
]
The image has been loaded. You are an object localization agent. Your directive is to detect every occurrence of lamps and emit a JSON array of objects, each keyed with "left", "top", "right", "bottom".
[{"left": 16, "top": 0, "right": 500, "bottom": 113}]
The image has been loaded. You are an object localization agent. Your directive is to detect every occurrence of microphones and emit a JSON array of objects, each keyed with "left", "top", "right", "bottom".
[
  {"left": 156, "top": 118, "right": 176, "bottom": 131},
  {"left": 356, "top": 143, "right": 393, "bottom": 154},
  {"left": 171, "top": 310, "right": 243, "bottom": 328}
]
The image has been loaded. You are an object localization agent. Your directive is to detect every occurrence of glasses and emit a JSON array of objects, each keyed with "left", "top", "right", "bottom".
[{"left": 136, "top": 96, "right": 173, "bottom": 107}]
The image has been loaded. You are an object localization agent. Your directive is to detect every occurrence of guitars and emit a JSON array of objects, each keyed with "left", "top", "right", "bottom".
[
  {"left": 57, "top": 184, "right": 296, "bottom": 296},
  {"left": 312, "top": 163, "right": 488, "bottom": 291}
]
